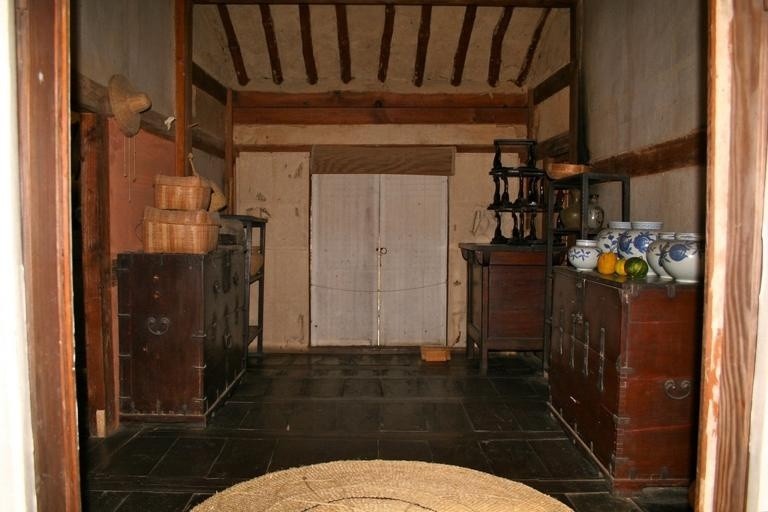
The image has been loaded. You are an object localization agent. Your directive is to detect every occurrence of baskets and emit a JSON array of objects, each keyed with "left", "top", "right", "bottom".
[
  {"left": 141, "top": 176, "right": 227, "bottom": 254},
  {"left": 421, "top": 346, "right": 451, "bottom": 362}
]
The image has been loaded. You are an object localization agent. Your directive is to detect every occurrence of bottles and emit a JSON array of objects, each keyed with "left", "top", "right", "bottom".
[
  {"left": 561, "top": 188, "right": 605, "bottom": 230},
  {"left": 568, "top": 221, "right": 704, "bottom": 287}
]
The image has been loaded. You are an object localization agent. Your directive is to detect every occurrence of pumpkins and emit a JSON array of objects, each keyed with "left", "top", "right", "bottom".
[
  {"left": 597, "top": 252, "right": 617, "bottom": 274},
  {"left": 625, "top": 257, "right": 648, "bottom": 278},
  {"left": 615, "top": 258, "right": 628, "bottom": 274}
]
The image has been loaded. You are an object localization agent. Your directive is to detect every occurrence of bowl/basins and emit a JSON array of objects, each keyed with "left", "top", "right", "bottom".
[{"left": 545, "top": 163, "right": 597, "bottom": 181}]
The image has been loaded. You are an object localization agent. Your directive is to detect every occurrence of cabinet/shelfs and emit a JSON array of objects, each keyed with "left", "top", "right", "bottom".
[
  {"left": 220, "top": 213, "right": 267, "bottom": 370},
  {"left": 544, "top": 265, "right": 702, "bottom": 498},
  {"left": 546, "top": 172, "right": 630, "bottom": 368},
  {"left": 118, "top": 252, "right": 251, "bottom": 428}
]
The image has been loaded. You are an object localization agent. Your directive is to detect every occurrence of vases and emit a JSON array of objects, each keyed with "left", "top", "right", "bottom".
[
  {"left": 558, "top": 183, "right": 603, "bottom": 229},
  {"left": 568, "top": 221, "right": 706, "bottom": 284}
]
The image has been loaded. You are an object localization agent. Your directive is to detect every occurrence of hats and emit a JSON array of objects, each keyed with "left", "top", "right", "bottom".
[{"left": 108, "top": 74, "right": 152, "bottom": 138}]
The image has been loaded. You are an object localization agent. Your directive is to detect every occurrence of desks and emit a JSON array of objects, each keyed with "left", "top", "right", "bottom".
[{"left": 458, "top": 243, "right": 550, "bottom": 370}]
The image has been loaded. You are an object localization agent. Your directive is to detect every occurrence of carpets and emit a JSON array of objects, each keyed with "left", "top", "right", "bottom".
[{"left": 188, "top": 459, "right": 576, "bottom": 512}]
